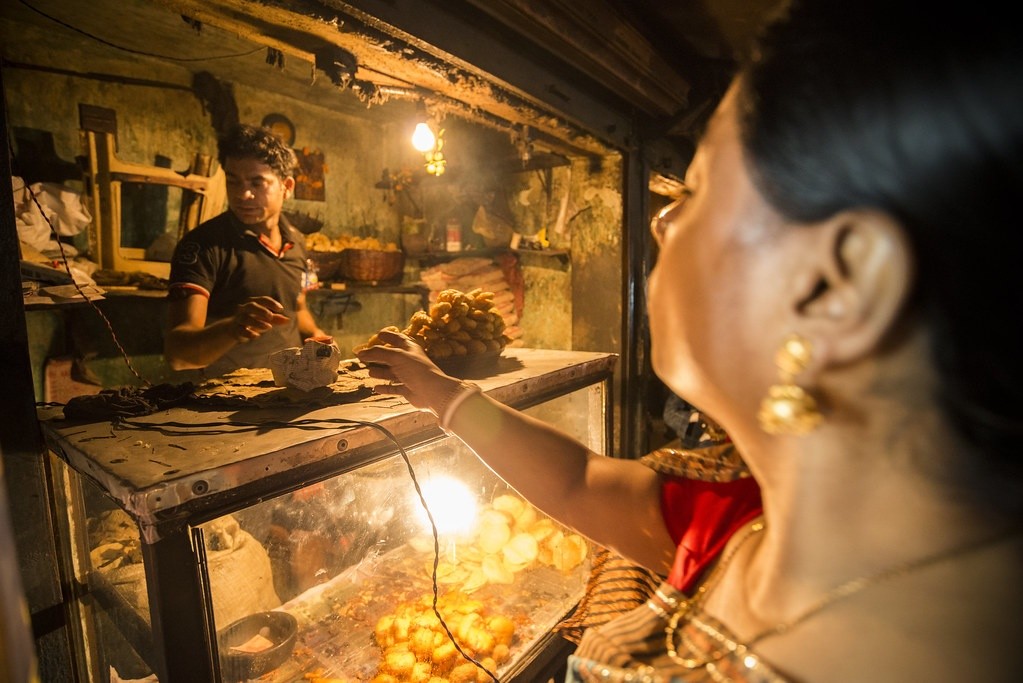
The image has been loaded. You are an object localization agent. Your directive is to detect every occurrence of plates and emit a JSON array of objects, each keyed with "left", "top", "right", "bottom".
[{"left": 354, "top": 345, "right": 504, "bottom": 372}]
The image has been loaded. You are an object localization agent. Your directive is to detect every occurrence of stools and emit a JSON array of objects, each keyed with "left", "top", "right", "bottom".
[{"left": 85, "top": 131, "right": 225, "bottom": 279}]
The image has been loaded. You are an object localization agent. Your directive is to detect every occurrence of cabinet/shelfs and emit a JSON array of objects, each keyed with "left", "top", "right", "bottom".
[{"left": 430, "top": 151, "right": 570, "bottom": 271}]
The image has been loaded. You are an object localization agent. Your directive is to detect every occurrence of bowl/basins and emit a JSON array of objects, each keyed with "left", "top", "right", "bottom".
[{"left": 217, "top": 611, "right": 298, "bottom": 680}]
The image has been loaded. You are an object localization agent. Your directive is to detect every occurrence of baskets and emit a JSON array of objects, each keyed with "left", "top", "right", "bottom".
[
  {"left": 308, "top": 251, "right": 340, "bottom": 281},
  {"left": 342, "top": 248, "right": 404, "bottom": 283}
]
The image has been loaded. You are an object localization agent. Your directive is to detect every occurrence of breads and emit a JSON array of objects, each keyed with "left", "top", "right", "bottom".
[
  {"left": 421, "top": 495, "right": 589, "bottom": 592},
  {"left": 306, "top": 231, "right": 398, "bottom": 252}
]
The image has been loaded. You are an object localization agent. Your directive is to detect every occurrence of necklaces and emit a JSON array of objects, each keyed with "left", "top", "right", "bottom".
[{"left": 665, "top": 518, "right": 1023, "bottom": 667}]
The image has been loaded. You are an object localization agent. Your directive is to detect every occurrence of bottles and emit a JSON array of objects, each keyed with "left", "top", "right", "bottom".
[{"left": 446, "top": 218, "right": 463, "bottom": 252}]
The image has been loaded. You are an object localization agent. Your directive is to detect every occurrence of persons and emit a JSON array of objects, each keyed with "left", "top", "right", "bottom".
[
  {"left": 359, "top": 0, "right": 1023, "bottom": 683},
  {"left": 162, "top": 124, "right": 330, "bottom": 382}
]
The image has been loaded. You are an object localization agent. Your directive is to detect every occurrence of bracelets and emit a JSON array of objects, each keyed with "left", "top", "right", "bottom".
[{"left": 438, "top": 380, "right": 484, "bottom": 437}]
